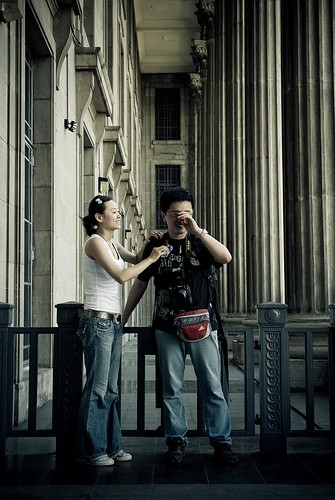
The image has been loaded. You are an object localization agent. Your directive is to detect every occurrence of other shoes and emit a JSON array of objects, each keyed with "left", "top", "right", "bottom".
[
  {"left": 109, "top": 449, "right": 132, "bottom": 461},
  {"left": 76, "top": 454, "right": 114, "bottom": 466},
  {"left": 165, "top": 437, "right": 186, "bottom": 463},
  {"left": 214, "top": 443, "right": 239, "bottom": 465}
]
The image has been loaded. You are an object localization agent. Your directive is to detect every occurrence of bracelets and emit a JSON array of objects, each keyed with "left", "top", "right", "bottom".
[{"left": 198, "top": 229, "right": 208, "bottom": 238}]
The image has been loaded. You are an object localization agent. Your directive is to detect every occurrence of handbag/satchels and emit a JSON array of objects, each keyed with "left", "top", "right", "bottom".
[{"left": 173, "top": 309, "right": 211, "bottom": 344}]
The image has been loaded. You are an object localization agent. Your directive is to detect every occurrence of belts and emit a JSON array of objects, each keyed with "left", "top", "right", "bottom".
[{"left": 82, "top": 309, "right": 122, "bottom": 325}]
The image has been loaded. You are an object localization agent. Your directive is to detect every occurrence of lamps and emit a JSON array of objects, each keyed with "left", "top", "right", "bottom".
[{"left": 63, "top": 117, "right": 77, "bottom": 133}]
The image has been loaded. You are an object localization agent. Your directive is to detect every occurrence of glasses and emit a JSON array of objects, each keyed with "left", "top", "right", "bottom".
[{"left": 165, "top": 212, "right": 180, "bottom": 217}]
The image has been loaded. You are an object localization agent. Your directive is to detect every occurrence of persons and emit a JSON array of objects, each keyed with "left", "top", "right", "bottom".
[
  {"left": 121, "top": 187, "right": 239, "bottom": 468},
  {"left": 77, "top": 195, "right": 167, "bottom": 466}
]
